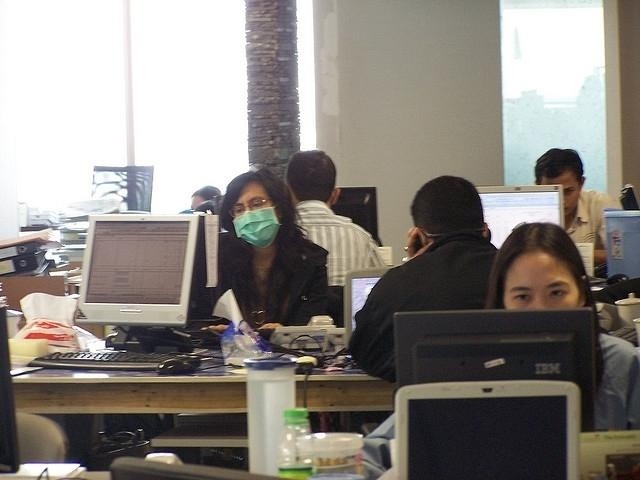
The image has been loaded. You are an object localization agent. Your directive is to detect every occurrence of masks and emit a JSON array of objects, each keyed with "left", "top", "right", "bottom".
[{"left": 232, "top": 206, "right": 283, "bottom": 249}]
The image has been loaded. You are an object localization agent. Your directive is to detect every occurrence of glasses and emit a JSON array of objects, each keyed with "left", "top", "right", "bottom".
[{"left": 228, "top": 198, "right": 274, "bottom": 217}]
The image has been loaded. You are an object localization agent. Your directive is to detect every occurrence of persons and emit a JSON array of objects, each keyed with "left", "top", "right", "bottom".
[
  {"left": 535, "top": 149, "right": 623, "bottom": 264},
  {"left": 348, "top": 175, "right": 497, "bottom": 384},
  {"left": 200, "top": 169, "right": 330, "bottom": 331},
  {"left": 191, "top": 186, "right": 221, "bottom": 215},
  {"left": 284, "top": 151, "right": 387, "bottom": 285},
  {"left": 361, "top": 222, "right": 640, "bottom": 480}
]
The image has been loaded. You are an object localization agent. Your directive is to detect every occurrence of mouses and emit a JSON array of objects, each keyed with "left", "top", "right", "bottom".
[{"left": 156, "top": 357, "right": 200, "bottom": 375}]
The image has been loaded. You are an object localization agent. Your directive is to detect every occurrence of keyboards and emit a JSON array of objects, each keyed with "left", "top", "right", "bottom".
[{"left": 28, "top": 352, "right": 175, "bottom": 370}]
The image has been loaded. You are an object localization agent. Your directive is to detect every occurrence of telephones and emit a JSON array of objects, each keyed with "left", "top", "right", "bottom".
[{"left": 270, "top": 315, "right": 346, "bottom": 351}]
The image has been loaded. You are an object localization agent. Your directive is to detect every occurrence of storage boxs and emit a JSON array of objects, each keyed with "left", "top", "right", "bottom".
[{"left": 602, "top": 206, "right": 640, "bottom": 283}]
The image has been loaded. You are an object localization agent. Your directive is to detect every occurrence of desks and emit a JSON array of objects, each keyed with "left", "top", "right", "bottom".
[{"left": 1, "top": 333, "right": 396, "bottom": 479}]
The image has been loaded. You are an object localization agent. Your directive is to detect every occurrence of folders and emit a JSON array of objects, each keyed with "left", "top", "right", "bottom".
[{"left": 0, "top": 242, "right": 55, "bottom": 278}]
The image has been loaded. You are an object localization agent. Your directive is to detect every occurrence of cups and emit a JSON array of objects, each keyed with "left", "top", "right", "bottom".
[
  {"left": 633, "top": 318, "right": 640, "bottom": 348},
  {"left": 615, "top": 293, "right": 640, "bottom": 329}
]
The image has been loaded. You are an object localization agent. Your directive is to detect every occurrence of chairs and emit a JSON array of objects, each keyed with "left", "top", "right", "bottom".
[{"left": 14, "top": 410, "right": 71, "bottom": 466}]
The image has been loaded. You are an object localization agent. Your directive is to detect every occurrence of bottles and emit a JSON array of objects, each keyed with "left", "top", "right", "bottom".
[
  {"left": 279, "top": 406, "right": 312, "bottom": 479},
  {"left": 243, "top": 357, "right": 297, "bottom": 476}
]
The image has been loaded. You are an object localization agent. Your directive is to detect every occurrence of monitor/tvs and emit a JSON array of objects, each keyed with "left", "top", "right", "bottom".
[
  {"left": 74, "top": 214, "right": 199, "bottom": 346},
  {"left": 394, "top": 379, "right": 582, "bottom": 480},
  {"left": 343, "top": 269, "right": 393, "bottom": 350},
  {"left": 331, "top": 186, "right": 379, "bottom": 243},
  {"left": 392, "top": 308, "right": 596, "bottom": 389},
  {"left": 476, "top": 184, "right": 565, "bottom": 249}
]
